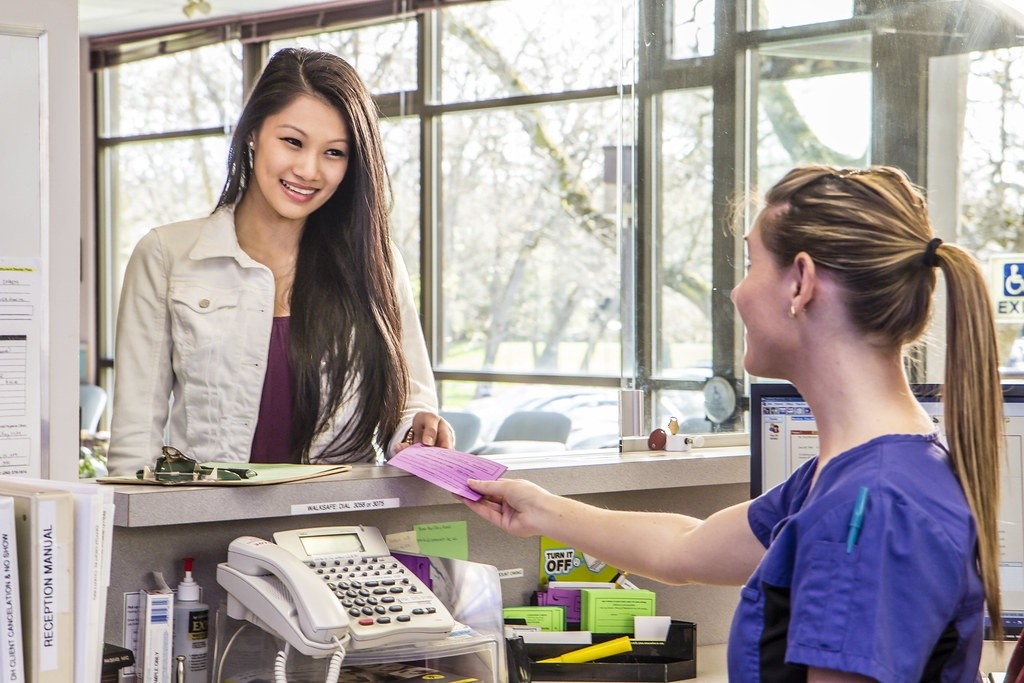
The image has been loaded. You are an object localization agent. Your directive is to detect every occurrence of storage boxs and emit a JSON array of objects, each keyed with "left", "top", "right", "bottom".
[{"left": 216, "top": 599, "right": 505, "bottom": 683}]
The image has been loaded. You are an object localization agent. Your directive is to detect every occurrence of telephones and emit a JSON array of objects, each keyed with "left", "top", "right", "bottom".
[{"left": 215, "top": 522, "right": 452, "bottom": 658}]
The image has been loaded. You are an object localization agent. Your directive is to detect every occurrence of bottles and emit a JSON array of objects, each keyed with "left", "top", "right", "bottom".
[{"left": 172, "top": 558, "right": 209, "bottom": 683}]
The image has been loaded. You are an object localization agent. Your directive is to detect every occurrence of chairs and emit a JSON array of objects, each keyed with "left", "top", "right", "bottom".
[
  {"left": 479, "top": 409, "right": 572, "bottom": 456},
  {"left": 80, "top": 384, "right": 108, "bottom": 432},
  {"left": 439, "top": 411, "right": 487, "bottom": 455}
]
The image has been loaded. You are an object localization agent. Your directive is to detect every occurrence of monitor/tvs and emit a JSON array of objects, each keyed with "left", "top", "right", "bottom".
[{"left": 750, "top": 382, "right": 1024, "bottom": 641}]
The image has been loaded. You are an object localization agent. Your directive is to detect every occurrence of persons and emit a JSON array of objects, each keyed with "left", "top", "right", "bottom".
[
  {"left": 451, "top": 159, "right": 1006, "bottom": 683},
  {"left": 105, "top": 47, "right": 458, "bottom": 479}
]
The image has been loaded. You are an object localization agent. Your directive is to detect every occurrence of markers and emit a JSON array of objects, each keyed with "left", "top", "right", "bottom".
[{"left": 536, "top": 635, "right": 633, "bottom": 664}]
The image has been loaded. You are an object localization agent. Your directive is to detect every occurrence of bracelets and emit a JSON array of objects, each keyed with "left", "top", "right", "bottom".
[{"left": 407, "top": 427, "right": 414, "bottom": 445}]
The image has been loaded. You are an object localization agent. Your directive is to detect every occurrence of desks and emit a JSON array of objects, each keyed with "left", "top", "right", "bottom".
[{"left": 77, "top": 448, "right": 1019, "bottom": 683}]
